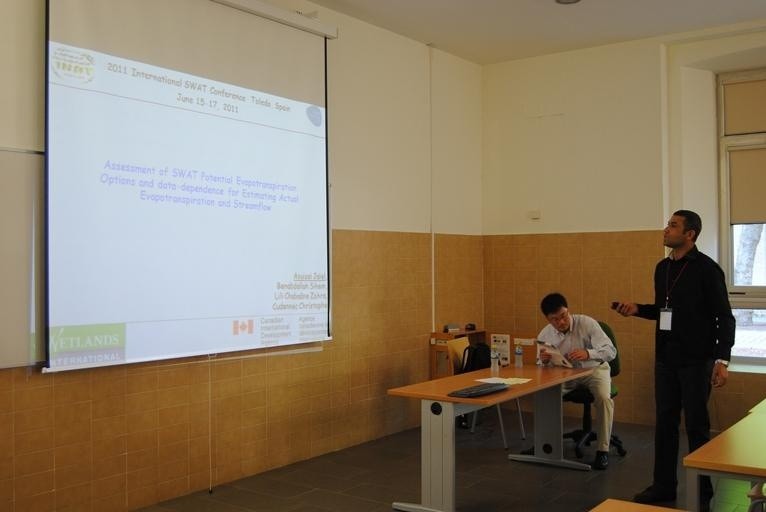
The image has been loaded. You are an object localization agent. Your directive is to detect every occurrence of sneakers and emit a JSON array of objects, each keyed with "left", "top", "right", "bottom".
[
  {"left": 520, "top": 443, "right": 553, "bottom": 455},
  {"left": 632, "top": 483, "right": 677, "bottom": 503},
  {"left": 593, "top": 450, "right": 608, "bottom": 470}
]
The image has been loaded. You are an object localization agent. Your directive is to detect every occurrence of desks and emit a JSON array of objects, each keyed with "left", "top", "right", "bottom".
[
  {"left": 387, "top": 363, "right": 593, "bottom": 512},
  {"left": 682, "top": 396, "right": 765, "bottom": 511}
]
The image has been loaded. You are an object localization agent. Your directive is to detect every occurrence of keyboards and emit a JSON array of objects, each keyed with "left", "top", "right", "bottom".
[{"left": 446, "top": 382, "right": 510, "bottom": 398}]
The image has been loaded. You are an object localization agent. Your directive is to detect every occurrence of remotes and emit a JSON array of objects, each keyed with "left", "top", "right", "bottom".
[{"left": 610, "top": 300, "right": 619, "bottom": 311}]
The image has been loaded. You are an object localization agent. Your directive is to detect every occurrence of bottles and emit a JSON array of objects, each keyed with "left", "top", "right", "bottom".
[
  {"left": 514, "top": 341, "right": 524, "bottom": 370},
  {"left": 491, "top": 345, "right": 500, "bottom": 374}
]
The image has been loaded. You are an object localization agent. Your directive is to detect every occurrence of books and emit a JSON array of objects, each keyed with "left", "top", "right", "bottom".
[{"left": 537, "top": 340, "right": 573, "bottom": 368}]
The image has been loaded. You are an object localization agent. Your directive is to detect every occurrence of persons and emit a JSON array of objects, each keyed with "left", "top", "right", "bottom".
[
  {"left": 612, "top": 209, "right": 738, "bottom": 505},
  {"left": 537, "top": 291, "right": 618, "bottom": 470}
]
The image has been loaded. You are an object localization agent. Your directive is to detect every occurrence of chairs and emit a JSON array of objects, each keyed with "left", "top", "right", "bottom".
[
  {"left": 562, "top": 320, "right": 627, "bottom": 458},
  {"left": 446, "top": 335, "right": 526, "bottom": 451}
]
